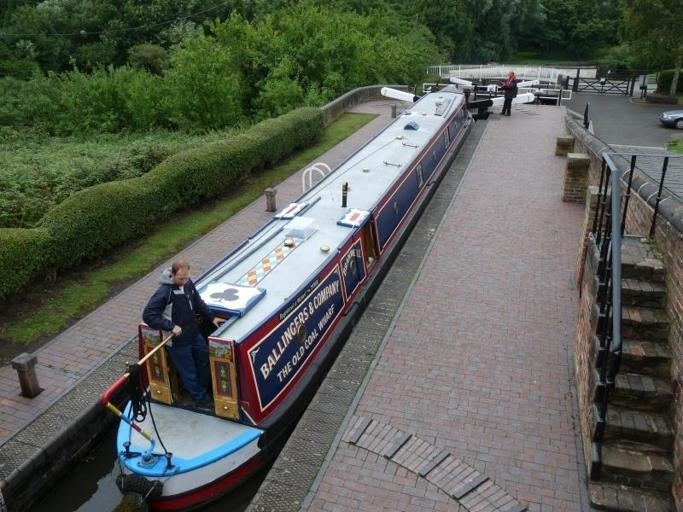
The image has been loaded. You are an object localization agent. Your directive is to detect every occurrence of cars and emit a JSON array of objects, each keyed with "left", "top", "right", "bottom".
[{"left": 656, "top": 107, "right": 682, "bottom": 131}]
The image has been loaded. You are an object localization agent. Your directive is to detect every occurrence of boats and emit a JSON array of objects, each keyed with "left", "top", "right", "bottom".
[{"left": 98, "top": 88, "right": 475, "bottom": 505}]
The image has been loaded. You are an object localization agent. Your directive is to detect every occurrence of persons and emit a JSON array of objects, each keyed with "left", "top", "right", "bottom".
[
  {"left": 499, "top": 70, "right": 518, "bottom": 116},
  {"left": 142, "top": 259, "right": 227, "bottom": 411}
]
